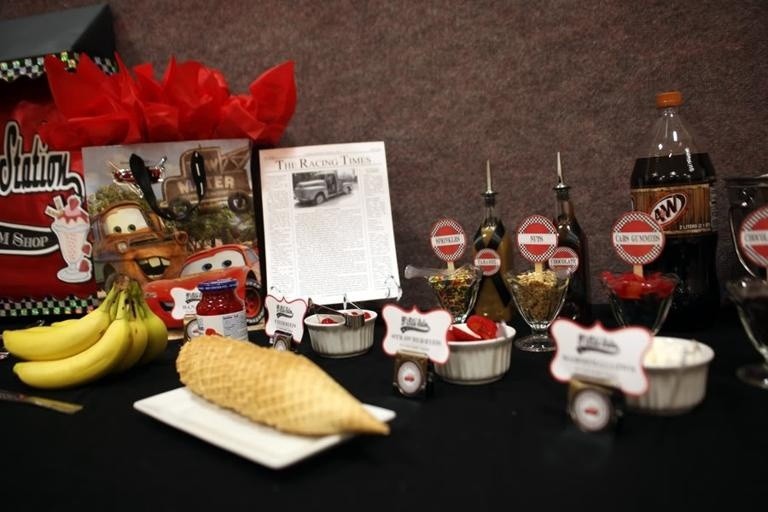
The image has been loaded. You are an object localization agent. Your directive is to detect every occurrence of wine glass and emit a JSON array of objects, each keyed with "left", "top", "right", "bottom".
[
  {"left": 426, "top": 268, "right": 569, "bottom": 351},
  {"left": 729, "top": 278, "right": 768, "bottom": 391}
]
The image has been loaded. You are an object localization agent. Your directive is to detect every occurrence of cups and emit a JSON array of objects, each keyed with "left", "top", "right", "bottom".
[
  {"left": 725, "top": 173, "right": 767, "bottom": 279},
  {"left": 602, "top": 275, "right": 677, "bottom": 337}
]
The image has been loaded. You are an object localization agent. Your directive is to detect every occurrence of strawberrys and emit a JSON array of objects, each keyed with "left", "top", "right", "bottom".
[{"left": 444, "top": 315, "right": 497, "bottom": 340}]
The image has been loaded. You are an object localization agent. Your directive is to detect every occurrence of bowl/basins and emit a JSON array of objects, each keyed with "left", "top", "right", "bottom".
[
  {"left": 305, "top": 308, "right": 379, "bottom": 358},
  {"left": 624, "top": 336, "right": 716, "bottom": 414},
  {"left": 431, "top": 324, "right": 514, "bottom": 384}
]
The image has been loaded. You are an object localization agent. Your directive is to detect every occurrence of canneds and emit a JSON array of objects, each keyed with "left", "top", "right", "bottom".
[{"left": 192, "top": 277, "right": 249, "bottom": 342}]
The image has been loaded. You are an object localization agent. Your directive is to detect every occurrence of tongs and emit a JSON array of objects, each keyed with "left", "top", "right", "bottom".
[{"left": 307, "top": 294, "right": 366, "bottom": 329}]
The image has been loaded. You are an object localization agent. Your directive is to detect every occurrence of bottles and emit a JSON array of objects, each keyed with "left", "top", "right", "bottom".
[
  {"left": 473, "top": 152, "right": 589, "bottom": 328},
  {"left": 630, "top": 87, "right": 722, "bottom": 323}
]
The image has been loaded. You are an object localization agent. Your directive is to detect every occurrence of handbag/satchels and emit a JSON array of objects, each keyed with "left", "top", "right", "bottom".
[{"left": 78, "top": 136, "right": 265, "bottom": 361}]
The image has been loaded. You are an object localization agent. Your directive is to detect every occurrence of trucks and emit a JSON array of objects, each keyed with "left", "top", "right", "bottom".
[{"left": 295, "top": 171, "right": 357, "bottom": 205}]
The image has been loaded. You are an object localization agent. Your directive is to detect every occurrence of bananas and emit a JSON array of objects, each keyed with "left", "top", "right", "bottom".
[{"left": 0, "top": 273, "right": 170, "bottom": 388}]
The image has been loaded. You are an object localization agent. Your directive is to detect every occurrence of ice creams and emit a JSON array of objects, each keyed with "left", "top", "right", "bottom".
[{"left": 45, "top": 194, "right": 91, "bottom": 272}]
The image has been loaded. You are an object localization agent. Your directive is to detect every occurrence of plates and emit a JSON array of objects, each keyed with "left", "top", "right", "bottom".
[{"left": 132, "top": 386, "right": 396, "bottom": 471}]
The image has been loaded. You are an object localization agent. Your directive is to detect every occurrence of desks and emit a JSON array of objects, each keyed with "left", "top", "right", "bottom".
[{"left": 0, "top": 304, "right": 767, "bottom": 511}]
